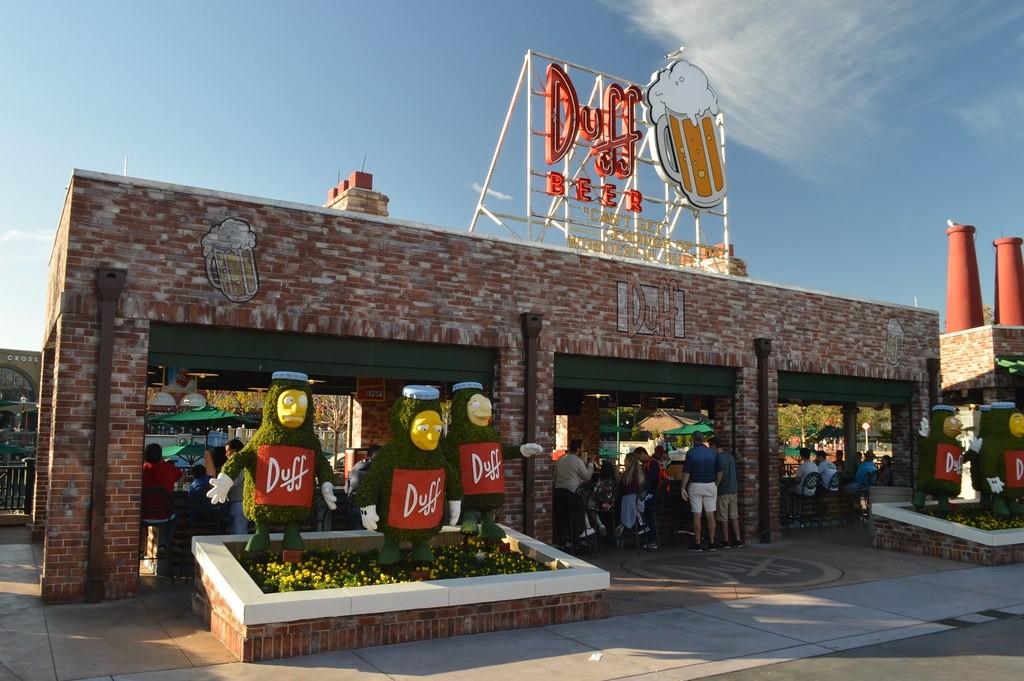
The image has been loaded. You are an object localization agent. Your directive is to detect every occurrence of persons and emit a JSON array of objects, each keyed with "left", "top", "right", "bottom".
[
  {"left": 707, "top": 437, "right": 744, "bottom": 549},
  {"left": 834, "top": 447, "right": 892, "bottom": 508},
  {"left": 140, "top": 443, "right": 184, "bottom": 547},
  {"left": 187, "top": 464, "right": 230, "bottom": 534},
  {"left": 635, "top": 447, "right": 663, "bottom": 548},
  {"left": 791, "top": 448, "right": 818, "bottom": 516},
  {"left": 554, "top": 439, "right": 594, "bottom": 551},
  {"left": 348, "top": 444, "right": 383, "bottom": 519},
  {"left": 615, "top": 453, "right": 644, "bottom": 537},
  {"left": 224, "top": 439, "right": 246, "bottom": 533},
  {"left": 817, "top": 450, "right": 839, "bottom": 506},
  {"left": 579, "top": 461, "right": 617, "bottom": 537},
  {"left": 681, "top": 431, "right": 723, "bottom": 552}
]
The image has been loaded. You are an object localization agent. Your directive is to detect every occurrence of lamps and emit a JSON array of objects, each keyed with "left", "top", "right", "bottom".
[
  {"left": 146, "top": 366, "right": 176, "bottom": 407},
  {"left": 186, "top": 368, "right": 218, "bottom": 378},
  {"left": 179, "top": 375, "right": 207, "bottom": 406}
]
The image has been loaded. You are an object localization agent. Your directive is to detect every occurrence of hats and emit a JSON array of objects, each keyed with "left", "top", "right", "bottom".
[{"left": 864, "top": 449, "right": 876, "bottom": 458}]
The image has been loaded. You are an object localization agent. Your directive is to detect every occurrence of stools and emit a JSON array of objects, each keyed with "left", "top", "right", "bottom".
[
  {"left": 140, "top": 487, "right": 178, "bottom": 575},
  {"left": 609, "top": 480, "right": 654, "bottom": 556},
  {"left": 784, "top": 470, "right": 873, "bottom": 532},
  {"left": 567, "top": 481, "right": 621, "bottom": 560},
  {"left": 639, "top": 478, "right": 670, "bottom": 552}
]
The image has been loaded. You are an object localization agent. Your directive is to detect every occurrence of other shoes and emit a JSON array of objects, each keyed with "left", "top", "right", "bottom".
[
  {"left": 158, "top": 545, "right": 167, "bottom": 552},
  {"left": 599, "top": 524, "right": 607, "bottom": 536},
  {"left": 638, "top": 524, "right": 650, "bottom": 535},
  {"left": 795, "top": 513, "right": 801, "bottom": 517},
  {"left": 709, "top": 543, "right": 716, "bottom": 552},
  {"left": 718, "top": 541, "right": 731, "bottom": 549},
  {"left": 643, "top": 543, "right": 658, "bottom": 548},
  {"left": 732, "top": 541, "right": 743, "bottom": 548},
  {"left": 688, "top": 544, "right": 702, "bottom": 552},
  {"left": 786, "top": 513, "right": 792, "bottom": 518},
  {"left": 579, "top": 528, "right": 595, "bottom": 537}
]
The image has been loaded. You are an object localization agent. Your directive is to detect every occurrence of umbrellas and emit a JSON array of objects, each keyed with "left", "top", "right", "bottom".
[
  {"left": 147, "top": 401, "right": 259, "bottom": 452},
  {"left": 600, "top": 447, "right": 620, "bottom": 455},
  {"left": 662, "top": 424, "right": 716, "bottom": 439},
  {"left": 599, "top": 421, "right": 634, "bottom": 433},
  {"left": 162, "top": 436, "right": 216, "bottom": 466}
]
